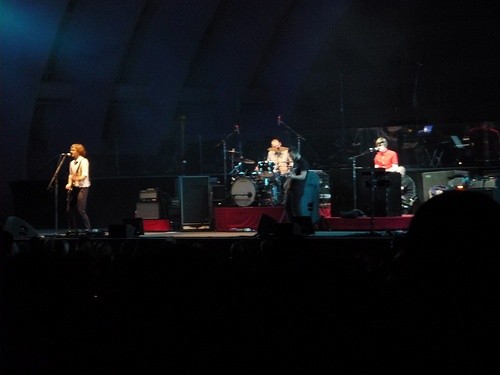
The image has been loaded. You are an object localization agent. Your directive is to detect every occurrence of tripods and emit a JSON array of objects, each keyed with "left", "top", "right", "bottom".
[{"left": 348, "top": 150, "right": 374, "bottom": 217}]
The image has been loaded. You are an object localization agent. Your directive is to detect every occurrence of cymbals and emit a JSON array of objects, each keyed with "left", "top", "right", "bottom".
[
  {"left": 234, "top": 156, "right": 256, "bottom": 165},
  {"left": 223, "top": 150, "right": 240, "bottom": 154},
  {"left": 266, "top": 146, "right": 290, "bottom": 152}
]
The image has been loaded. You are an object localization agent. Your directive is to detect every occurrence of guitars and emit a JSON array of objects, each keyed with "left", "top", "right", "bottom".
[
  {"left": 281, "top": 173, "right": 292, "bottom": 207},
  {"left": 62, "top": 166, "right": 77, "bottom": 206}
]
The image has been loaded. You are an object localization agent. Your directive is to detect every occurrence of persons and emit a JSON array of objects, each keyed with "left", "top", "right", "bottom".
[
  {"left": 0, "top": 189, "right": 500, "bottom": 375},
  {"left": 65, "top": 144, "right": 91, "bottom": 229},
  {"left": 267, "top": 139, "right": 293, "bottom": 176},
  {"left": 283, "top": 146, "right": 309, "bottom": 215},
  {"left": 397, "top": 166, "right": 415, "bottom": 204},
  {"left": 374, "top": 137, "right": 398, "bottom": 172}
]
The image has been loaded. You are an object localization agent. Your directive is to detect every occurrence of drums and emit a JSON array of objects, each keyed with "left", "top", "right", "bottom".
[
  {"left": 229, "top": 175, "right": 257, "bottom": 207},
  {"left": 256, "top": 185, "right": 278, "bottom": 203},
  {"left": 257, "top": 160, "right": 277, "bottom": 178}
]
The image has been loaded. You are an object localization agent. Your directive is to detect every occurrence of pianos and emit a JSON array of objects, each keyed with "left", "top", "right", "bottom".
[{"left": 330, "top": 167, "right": 403, "bottom": 218}]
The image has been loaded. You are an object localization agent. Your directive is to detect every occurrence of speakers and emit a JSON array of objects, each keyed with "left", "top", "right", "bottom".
[
  {"left": 176, "top": 176, "right": 226, "bottom": 226},
  {"left": 3, "top": 217, "right": 38, "bottom": 238},
  {"left": 108, "top": 201, "right": 168, "bottom": 238},
  {"left": 255, "top": 213, "right": 315, "bottom": 237},
  {"left": 420, "top": 171, "right": 453, "bottom": 205}
]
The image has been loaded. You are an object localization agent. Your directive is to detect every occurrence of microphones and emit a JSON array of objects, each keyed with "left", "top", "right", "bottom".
[
  {"left": 277, "top": 115, "right": 280, "bottom": 126},
  {"left": 62, "top": 153, "right": 71, "bottom": 156},
  {"left": 369, "top": 147, "right": 378, "bottom": 151},
  {"left": 248, "top": 193, "right": 251, "bottom": 197},
  {"left": 236, "top": 125, "right": 239, "bottom": 134}
]
